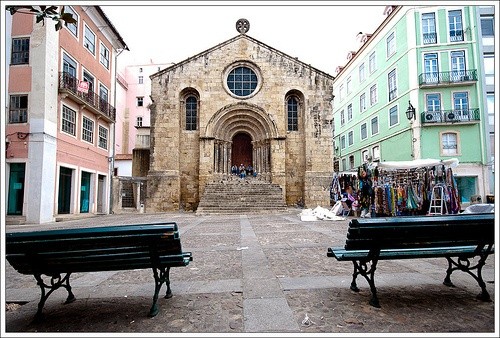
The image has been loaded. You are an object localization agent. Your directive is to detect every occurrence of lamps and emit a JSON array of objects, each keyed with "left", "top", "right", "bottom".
[{"left": 405, "top": 99, "right": 416, "bottom": 121}]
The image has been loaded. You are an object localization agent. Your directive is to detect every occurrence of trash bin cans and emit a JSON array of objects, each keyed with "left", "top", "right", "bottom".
[{"left": 140, "top": 204, "right": 145, "bottom": 213}]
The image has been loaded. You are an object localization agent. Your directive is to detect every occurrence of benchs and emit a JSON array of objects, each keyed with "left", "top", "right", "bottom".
[
  {"left": 5, "top": 221, "right": 195, "bottom": 318},
  {"left": 326, "top": 212, "right": 495, "bottom": 310}
]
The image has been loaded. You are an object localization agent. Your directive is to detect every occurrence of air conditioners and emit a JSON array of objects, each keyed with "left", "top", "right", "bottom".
[
  {"left": 444, "top": 111, "right": 459, "bottom": 122},
  {"left": 334, "top": 145, "right": 338, "bottom": 150},
  {"left": 422, "top": 111, "right": 438, "bottom": 123},
  {"left": 365, "top": 154, "right": 372, "bottom": 160}
]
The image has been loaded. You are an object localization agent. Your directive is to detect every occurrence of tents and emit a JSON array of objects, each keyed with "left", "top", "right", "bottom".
[{"left": 366, "top": 158, "right": 463, "bottom": 219}]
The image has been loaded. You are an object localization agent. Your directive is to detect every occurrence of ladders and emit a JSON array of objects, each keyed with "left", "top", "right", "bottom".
[{"left": 427, "top": 185, "right": 449, "bottom": 215}]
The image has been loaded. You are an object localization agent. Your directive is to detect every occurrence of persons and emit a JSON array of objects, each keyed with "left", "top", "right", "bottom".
[{"left": 230, "top": 163, "right": 254, "bottom": 179}]
